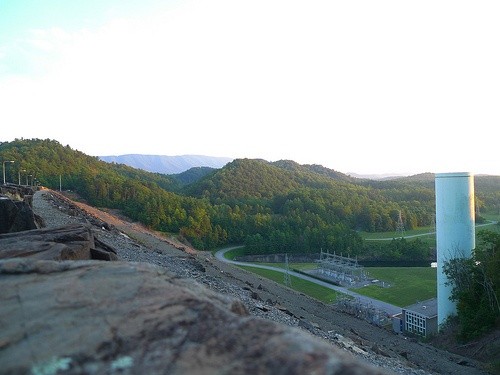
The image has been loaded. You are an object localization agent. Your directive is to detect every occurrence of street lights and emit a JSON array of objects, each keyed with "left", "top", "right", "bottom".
[
  {"left": 26, "top": 174, "right": 32, "bottom": 186},
  {"left": 18, "top": 170, "right": 26, "bottom": 186},
  {"left": 30, "top": 178, "right": 41, "bottom": 187},
  {"left": 3, "top": 160, "right": 15, "bottom": 184}
]
